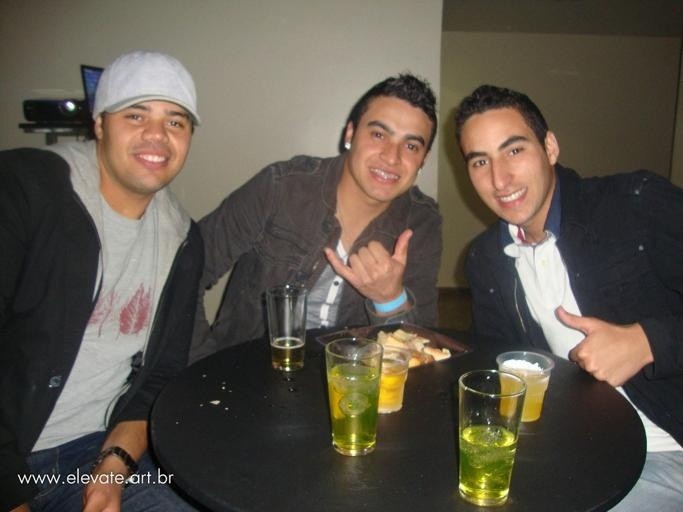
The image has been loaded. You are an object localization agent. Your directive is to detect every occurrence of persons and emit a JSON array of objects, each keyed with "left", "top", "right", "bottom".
[
  {"left": 0, "top": 51, "right": 205, "bottom": 512},
  {"left": 453, "top": 84, "right": 682, "bottom": 512},
  {"left": 186, "top": 74, "right": 443, "bottom": 367}
]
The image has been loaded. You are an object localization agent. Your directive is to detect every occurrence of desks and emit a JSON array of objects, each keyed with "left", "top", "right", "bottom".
[{"left": 148, "top": 325, "right": 647, "bottom": 512}]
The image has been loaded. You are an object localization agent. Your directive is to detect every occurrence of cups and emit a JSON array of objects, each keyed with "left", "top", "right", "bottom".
[
  {"left": 323, "top": 336, "right": 385, "bottom": 458},
  {"left": 374, "top": 348, "right": 411, "bottom": 415},
  {"left": 494, "top": 350, "right": 557, "bottom": 423},
  {"left": 455, "top": 368, "right": 530, "bottom": 508},
  {"left": 262, "top": 284, "right": 308, "bottom": 372}
]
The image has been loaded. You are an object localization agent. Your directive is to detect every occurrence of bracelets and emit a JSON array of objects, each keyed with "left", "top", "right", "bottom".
[{"left": 372, "top": 289, "right": 408, "bottom": 313}]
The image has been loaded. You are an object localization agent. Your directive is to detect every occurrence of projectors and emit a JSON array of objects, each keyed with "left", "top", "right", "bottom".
[{"left": 22, "top": 99, "right": 88, "bottom": 123}]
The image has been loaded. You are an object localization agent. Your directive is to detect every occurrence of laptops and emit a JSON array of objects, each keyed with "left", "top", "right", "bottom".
[{"left": 80, "top": 64, "right": 106, "bottom": 140}]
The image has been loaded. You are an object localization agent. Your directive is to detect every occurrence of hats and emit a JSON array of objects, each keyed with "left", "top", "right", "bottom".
[{"left": 94, "top": 51, "right": 201, "bottom": 127}]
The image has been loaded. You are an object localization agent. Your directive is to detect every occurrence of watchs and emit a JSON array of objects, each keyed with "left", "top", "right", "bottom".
[{"left": 92, "top": 445, "right": 143, "bottom": 490}]
